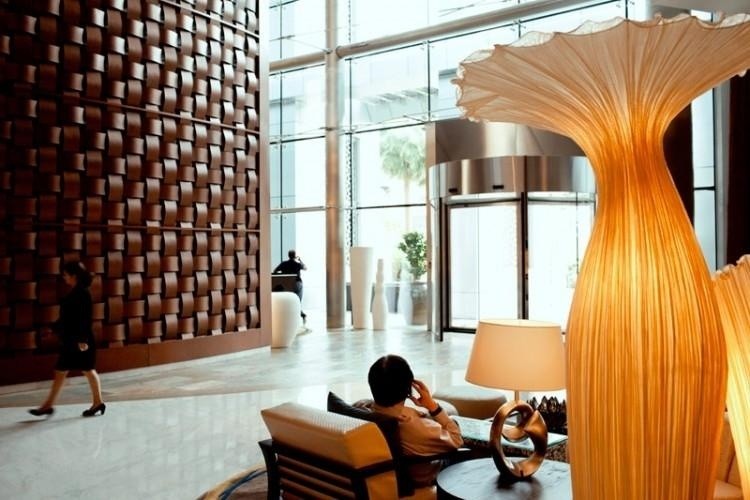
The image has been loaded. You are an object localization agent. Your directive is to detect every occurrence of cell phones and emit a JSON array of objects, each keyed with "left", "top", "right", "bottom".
[{"left": 408, "top": 375, "right": 414, "bottom": 398}]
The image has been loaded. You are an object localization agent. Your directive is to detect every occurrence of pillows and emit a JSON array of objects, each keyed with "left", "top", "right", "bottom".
[{"left": 328, "top": 389, "right": 416, "bottom": 498}]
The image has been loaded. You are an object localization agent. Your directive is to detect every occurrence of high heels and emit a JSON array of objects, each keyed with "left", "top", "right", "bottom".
[
  {"left": 81, "top": 403, "right": 106, "bottom": 418},
  {"left": 28, "top": 407, "right": 54, "bottom": 416}
]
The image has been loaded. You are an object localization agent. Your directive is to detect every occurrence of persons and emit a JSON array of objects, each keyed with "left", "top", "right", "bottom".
[
  {"left": 272, "top": 247, "right": 310, "bottom": 326},
  {"left": 28, "top": 259, "right": 106, "bottom": 420},
  {"left": 345, "top": 342, "right": 468, "bottom": 483}
]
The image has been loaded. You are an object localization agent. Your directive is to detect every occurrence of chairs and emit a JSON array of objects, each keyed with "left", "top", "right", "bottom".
[{"left": 258, "top": 438, "right": 461, "bottom": 500}]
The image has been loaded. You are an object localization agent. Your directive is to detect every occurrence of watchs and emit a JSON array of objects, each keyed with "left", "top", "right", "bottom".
[{"left": 428, "top": 404, "right": 442, "bottom": 417}]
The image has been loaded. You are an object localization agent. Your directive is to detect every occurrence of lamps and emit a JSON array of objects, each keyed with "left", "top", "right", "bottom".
[{"left": 463, "top": 319, "right": 567, "bottom": 478}]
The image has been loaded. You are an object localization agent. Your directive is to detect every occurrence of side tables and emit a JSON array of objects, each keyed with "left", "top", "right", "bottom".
[{"left": 437, "top": 456, "right": 572, "bottom": 500}]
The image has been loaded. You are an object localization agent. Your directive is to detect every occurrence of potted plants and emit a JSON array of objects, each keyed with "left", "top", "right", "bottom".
[{"left": 397, "top": 230, "right": 427, "bottom": 327}]
